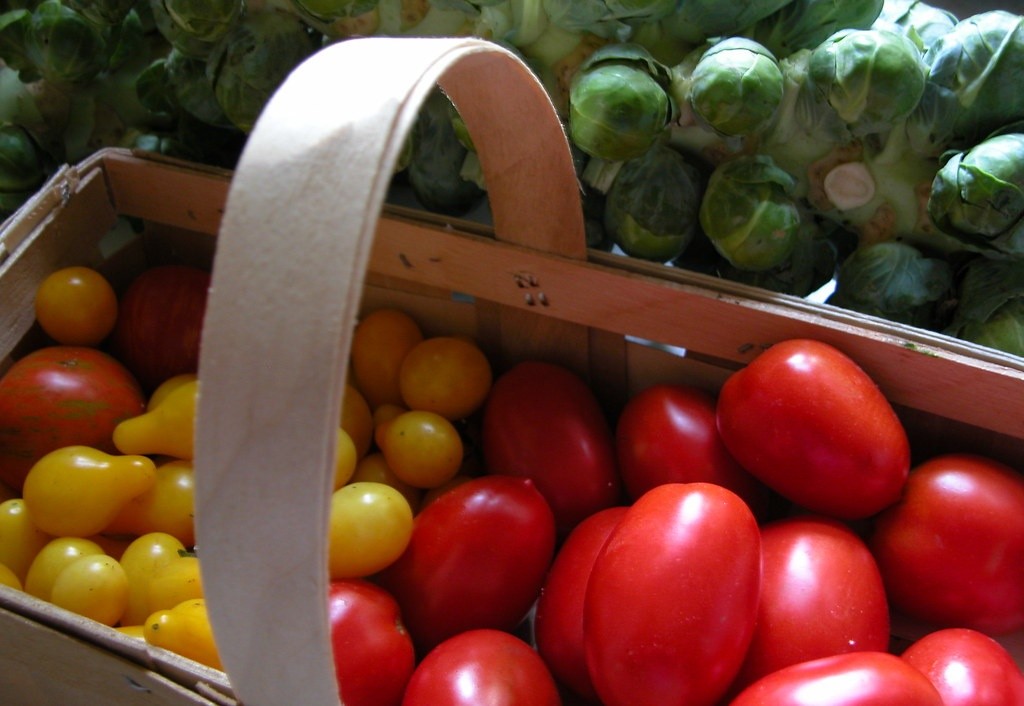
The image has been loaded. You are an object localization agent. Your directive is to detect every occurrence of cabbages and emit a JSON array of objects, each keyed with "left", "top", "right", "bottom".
[{"left": 0, "top": 0, "right": 1024, "bottom": 358}]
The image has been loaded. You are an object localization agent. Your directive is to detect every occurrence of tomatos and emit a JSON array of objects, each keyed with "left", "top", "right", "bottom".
[{"left": 0, "top": 251, "right": 1024, "bottom": 706}]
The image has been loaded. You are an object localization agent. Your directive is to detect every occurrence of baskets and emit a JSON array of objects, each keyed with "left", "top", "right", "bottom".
[{"left": 0, "top": 38, "right": 1024, "bottom": 706}]
